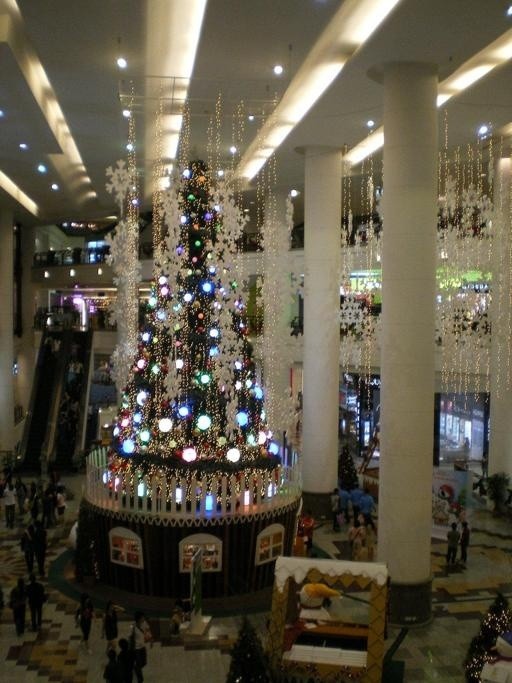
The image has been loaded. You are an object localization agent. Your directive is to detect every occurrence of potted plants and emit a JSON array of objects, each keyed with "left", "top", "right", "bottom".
[{"left": 473, "top": 471, "right": 511, "bottom": 519}]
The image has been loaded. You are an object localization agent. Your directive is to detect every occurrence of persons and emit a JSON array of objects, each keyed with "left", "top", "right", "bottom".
[
  {"left": 59, "top": 360, "right": 84, "bottom": 441},
  {"left": 44, "top": 334, "right": 60, "bottom": 353},
  {"left": 75, "top": 593, "right": 147, "bottom": 683},
  {"left": 329, "top": 481, "right": 377, "bottom": 559},
  {"left": 463, "top": 437, "right": 469, "bottom": 447},
  {"left": 300, "top": 508, "right": 315, "bottom": 557},
  {"left": 3, "top": 472, "right": 65, "bottom": 529},
  {"left": 447, "top": 429, "right": 453, "bottom": 442},
  {"left": 10, "top": 520, "right": 47, "bottom": 636},
  {"left": 446, "top": 521, "right": 470, "bottom": 563}
]
